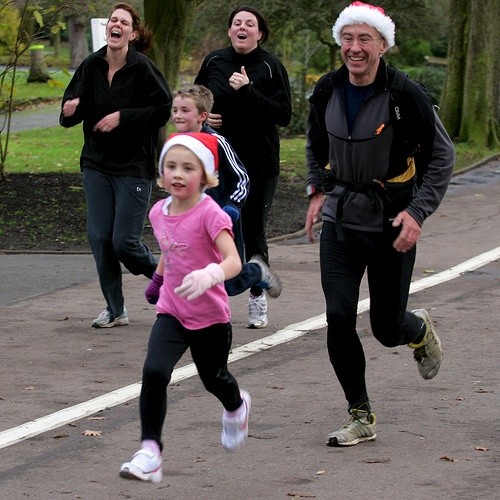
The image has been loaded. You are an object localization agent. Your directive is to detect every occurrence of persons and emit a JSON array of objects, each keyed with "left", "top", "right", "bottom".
[
  {"left": 119, "top": 132, "right": 252, "bottom": 483},
  {"left": 59, "top": 2, "right": 173, "bottom": 326},
  {"left": 172, "top": 85, "right": 281, "bottom": 299},
  {"left": 192, "top": 5, "right": 292, "bottom": 328},
  {"left": 306, "top": 1, "right": 456, "bottom": 447}
]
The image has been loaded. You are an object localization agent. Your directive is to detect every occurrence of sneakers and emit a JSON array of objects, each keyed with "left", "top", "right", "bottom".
[
  {"left": 408, "top": 308, "right": 442, "bottom": 379},
  {"left": 328, "top": 409, "right": 377, "bottom": 446},
  {"left": 248, "top": 254, "right": 283, "bottom": 299},
  {"left": 91, "top": 306, "right": 130, "bottom": 328},
  {"left": 248, "top": 291, "right": 268, "bottom": 328},
  {"left": 219, "top": 390, "right": 252, "bottom": 451},
  {"left": 120, "top": 448, "right": 163, "bottom": 484}
]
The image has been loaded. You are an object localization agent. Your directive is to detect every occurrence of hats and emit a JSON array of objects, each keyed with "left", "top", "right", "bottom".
[
  {"left": 156, "top": 133, "right": 220, "bottom": 189},
  {"left": 331, "top": 2, "right": 396, "bottom": 51}
]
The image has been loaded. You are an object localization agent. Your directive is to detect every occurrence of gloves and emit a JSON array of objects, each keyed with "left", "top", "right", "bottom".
[
  {"left": 175, "top": 263, "right": 225, "bottom": 301},
  {"left": 222, "top": 204, "right": 240, "bottom": 222},
  {"left": 145, "top": 271, "right": 164, "bottom": 304}
]
